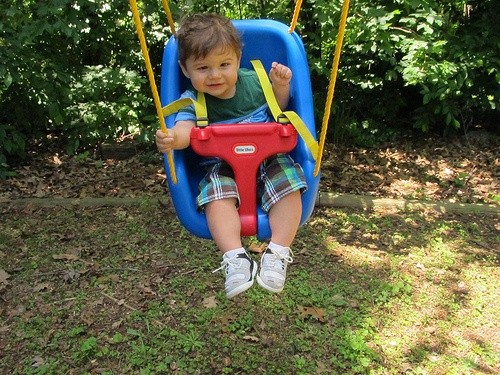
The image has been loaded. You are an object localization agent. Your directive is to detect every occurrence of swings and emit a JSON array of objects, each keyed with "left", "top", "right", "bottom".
[{"left": 131, "top": 0, "right": 350, "bottom": 241}]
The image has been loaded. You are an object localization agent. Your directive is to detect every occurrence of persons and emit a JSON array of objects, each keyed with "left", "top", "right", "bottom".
[{"left": 156, "top": 14, "right": 308, "bottom": 299}]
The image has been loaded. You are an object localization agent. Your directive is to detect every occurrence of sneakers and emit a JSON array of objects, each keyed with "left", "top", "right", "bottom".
[
  {"left": 256, "top": 246, "right": 294, "bottom": 293},
  {"left": 212, "top": 251, "right": 257, "bottom": 298}
]
